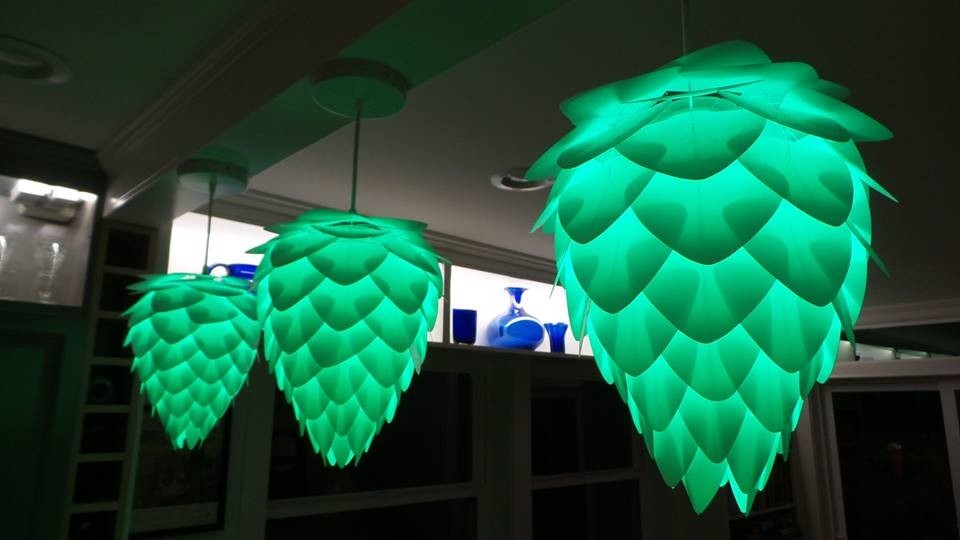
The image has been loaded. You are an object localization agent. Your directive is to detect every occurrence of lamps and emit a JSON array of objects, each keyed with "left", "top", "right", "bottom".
[
  {"left": 525, "top": 0, "right": 899, "bottom": 515},
  {"left": 246, "top": 58, "right": 445, "bottom": 468},
  {"left": 119, "top": 159, "right": 262, "bottom": 450}
]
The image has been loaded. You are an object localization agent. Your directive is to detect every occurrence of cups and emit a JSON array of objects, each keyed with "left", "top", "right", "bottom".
[
  {"left": 452, "top": 308, "right": 477, "bottom": 345},
  {"left": 543, "top": 322, "right": 569, "bottom": 352}
]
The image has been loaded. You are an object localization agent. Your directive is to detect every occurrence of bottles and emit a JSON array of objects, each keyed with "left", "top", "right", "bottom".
[{"left": 487, "top": 286, "right": 544, "bottom": 351}]
